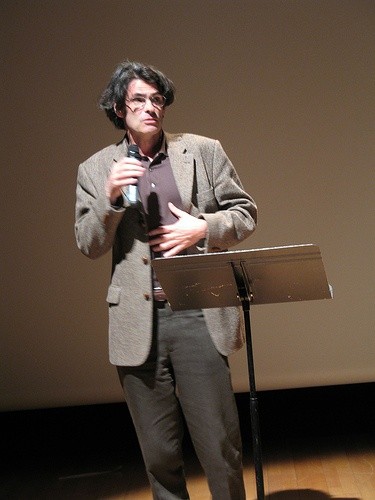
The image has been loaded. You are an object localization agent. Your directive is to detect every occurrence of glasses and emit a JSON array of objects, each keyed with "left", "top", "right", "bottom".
[{"left": 123, "top": 96, "right": 166, "bottom": 108}]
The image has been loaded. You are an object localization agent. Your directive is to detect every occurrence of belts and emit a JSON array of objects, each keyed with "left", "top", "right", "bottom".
[{"left": 152, "top": 287, "right": 168, "bottom": 301}]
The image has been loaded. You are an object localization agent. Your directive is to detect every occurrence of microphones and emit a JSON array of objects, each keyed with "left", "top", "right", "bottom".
[{"left": 126, "top": 144, "right": 139, "bottom": 207}]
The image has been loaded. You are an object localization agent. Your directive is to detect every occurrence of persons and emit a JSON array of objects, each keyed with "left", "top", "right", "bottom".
[{"left": 70, "top": 64, "right": 260, "bottom": 499}]
